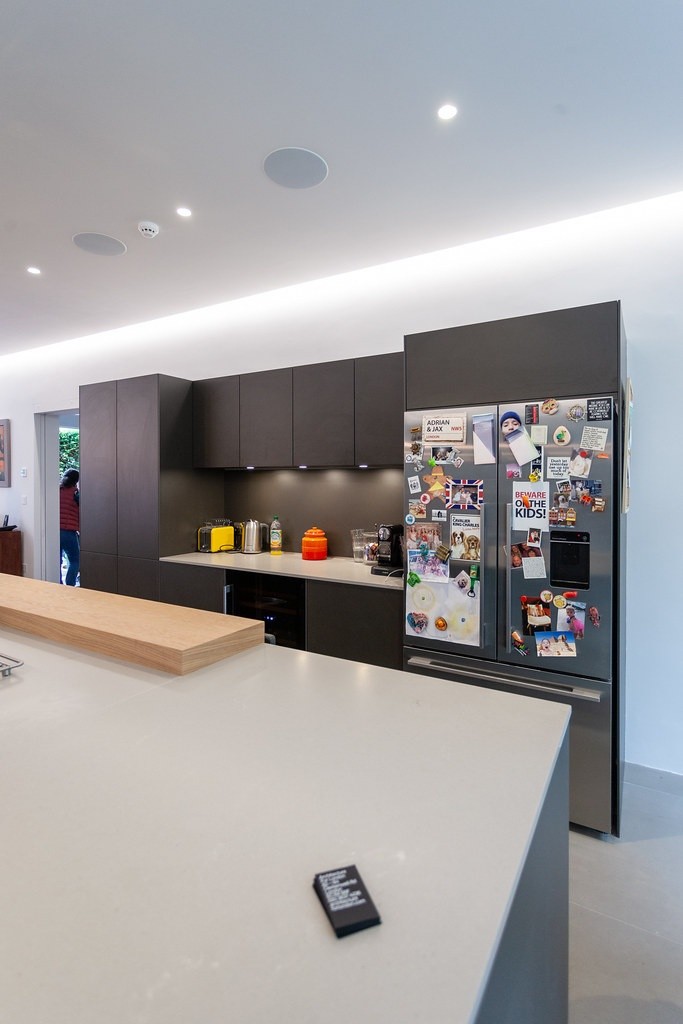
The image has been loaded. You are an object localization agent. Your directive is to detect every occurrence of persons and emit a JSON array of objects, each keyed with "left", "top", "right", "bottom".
[
  {"left": 507, "top": 526, "right": 547, "bottom": 567},
  {"left": 437, "top": 511, "right": 444, "bottom": 518},
  {"left": 565, "top": 606, "right": 583, "bottom": 640},
  {"left": 538, "top": 638, "right": 559, "bottom": 656},
  {"left": 408, "top": 524, "right": 440, "bottom": 548},
  {"left": 60, "top": 468, "right": 80, "bottom": 586},
  {"left": 432, "top": 450, "right": 450, "bottom": 461},
  {"left": 453, "top": 487, "right": 476, "bottom": 504},
  {"left": 500, "top": 410, "right": 522, "bottom": 441}
]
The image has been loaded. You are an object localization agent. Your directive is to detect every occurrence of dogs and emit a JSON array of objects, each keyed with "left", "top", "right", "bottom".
[{"left": 451, "top": 531, "right": 480, "bottom": 561}]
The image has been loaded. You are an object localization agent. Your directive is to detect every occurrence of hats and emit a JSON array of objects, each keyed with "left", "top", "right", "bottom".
[{"left": 500, "top": 412, "right": 521, "bottom": 426}]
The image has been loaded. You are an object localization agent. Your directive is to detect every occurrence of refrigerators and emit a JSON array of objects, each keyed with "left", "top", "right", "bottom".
[{"left": 405, "top": 396, "right": 617, "bottom": 836}]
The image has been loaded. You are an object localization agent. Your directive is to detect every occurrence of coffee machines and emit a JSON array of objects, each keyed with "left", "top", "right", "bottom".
[{"left": 371, "top": 525, "right": 404, "bottom": 577}]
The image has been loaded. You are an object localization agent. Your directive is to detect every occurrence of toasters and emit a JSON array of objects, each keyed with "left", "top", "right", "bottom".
[{"left": 198, "top": 519, "right": 234, "bottom": 552}]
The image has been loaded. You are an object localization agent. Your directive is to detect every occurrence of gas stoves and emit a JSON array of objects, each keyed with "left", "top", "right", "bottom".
[{"left": 0, "top": 652, "right": 22, "bottom": 677}]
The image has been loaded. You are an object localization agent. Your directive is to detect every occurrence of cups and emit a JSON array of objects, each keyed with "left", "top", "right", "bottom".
[{"left": 351, "top": 529, "right": 378, "bottom": 564}]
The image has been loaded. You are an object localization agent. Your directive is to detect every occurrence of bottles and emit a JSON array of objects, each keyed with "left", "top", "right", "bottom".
[
  {"left": 302, "top": 527, "right": 327, "bottom": 560},
  {"left": 270, "top": 516, "right": 282, "bottom": 555}
]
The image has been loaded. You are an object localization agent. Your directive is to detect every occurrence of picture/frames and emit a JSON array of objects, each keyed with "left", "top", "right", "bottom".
[{"left": 0, "top": 419, "right": 12, "bottom": 488}]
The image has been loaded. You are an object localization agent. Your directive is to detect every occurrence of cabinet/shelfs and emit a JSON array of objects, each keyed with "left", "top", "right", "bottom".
[
  {"left": 192, "top": 351, "right": 404, "bottom": 470},
  {"left": 307, "top": 579, "right": 405, "bottom": 669},
  {"left": 0, "top": 530, "right": 22, "bottom": 575},
  {"left": 78, "top": 373, "right": 227, "bottom": 614}
]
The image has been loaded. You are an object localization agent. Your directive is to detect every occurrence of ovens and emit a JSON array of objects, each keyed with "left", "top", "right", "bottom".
[{"left": 224, "top": 569, "right": 306, "bottom": 652}]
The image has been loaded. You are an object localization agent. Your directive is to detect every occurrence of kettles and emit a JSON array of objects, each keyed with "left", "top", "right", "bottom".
[{"left": 241, "top": 519, "right": 269, "bottom": 553}]
